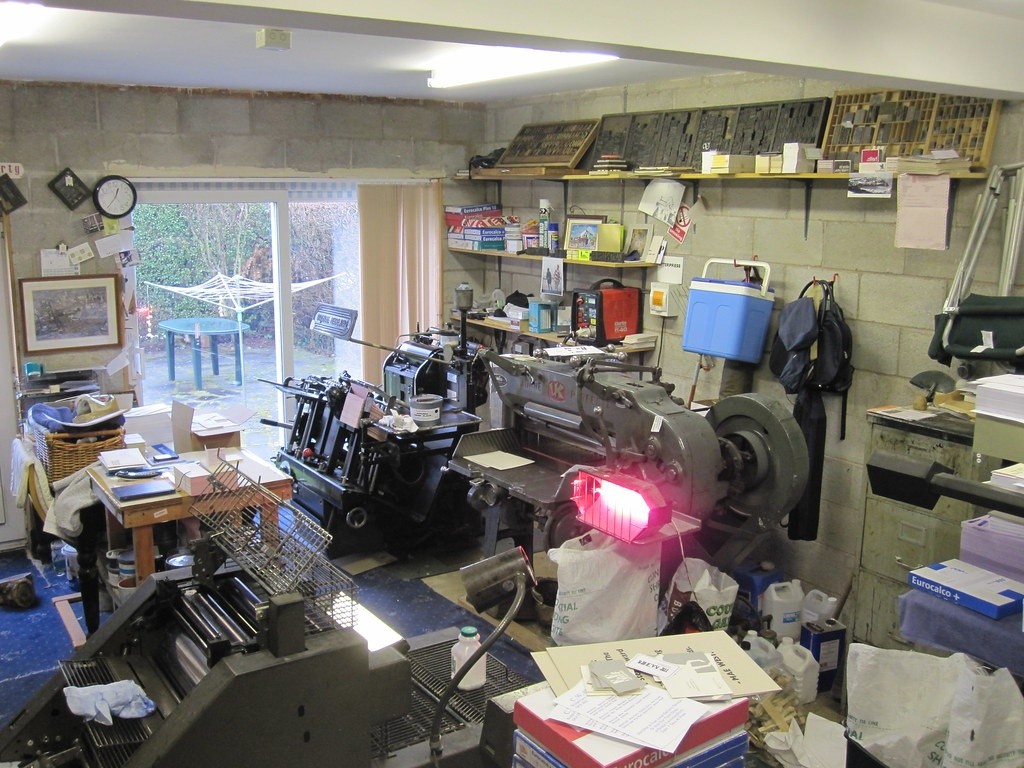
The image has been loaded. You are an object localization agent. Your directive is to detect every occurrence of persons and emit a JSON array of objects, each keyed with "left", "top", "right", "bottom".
[
  {"left": 553, "top": 265, "right": 561, "bottom": 292},
  {"left": 545, "top": 268, "right": 552, "bottom": 291}
]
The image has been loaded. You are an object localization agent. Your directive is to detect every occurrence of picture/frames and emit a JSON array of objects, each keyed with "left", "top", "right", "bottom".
[
  {"left": 562, "top": 214, "right": 607, "bottom": 252},
  {"left": 17, "top": 271, "right": 126, "bottom": 358}
]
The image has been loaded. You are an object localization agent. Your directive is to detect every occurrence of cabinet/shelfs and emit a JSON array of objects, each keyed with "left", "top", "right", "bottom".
[{"left": 445, "top": 246, "right": 658, "bottom": 358}]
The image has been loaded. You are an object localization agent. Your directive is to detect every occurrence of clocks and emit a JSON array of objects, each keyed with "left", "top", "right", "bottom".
[{"left": 92, "top": 175, "right": 137, "bottom": 219}]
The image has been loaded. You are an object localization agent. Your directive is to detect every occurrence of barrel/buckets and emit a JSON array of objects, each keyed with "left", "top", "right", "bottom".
[
  {"left": 50, "top": 538, "right": 83, "bottom": 592},
  {"left": 105, "top": 547, "right": 195, "bottom": 605}
]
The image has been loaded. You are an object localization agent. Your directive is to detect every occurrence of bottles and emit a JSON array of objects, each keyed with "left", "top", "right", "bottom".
[
  {"left": 522, "top": 199, "right": 559, "bottom": 254},
  {"left": 491, "top": 287, "right": 506, "bottom": 309},
  {"left": 723, "top": 555, "right": 838, "bottom": 706},
  {"left": 450, "top": 626, "right": 487, "bottom": 691}
]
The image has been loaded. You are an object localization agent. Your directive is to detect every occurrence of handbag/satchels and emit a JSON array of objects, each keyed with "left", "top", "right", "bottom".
[
  {"left": 548, "top": 529, "right": 661, "bottom": 646},
  {"left": 770, "top": 281, "right": 852, "bottom": 395},
  {"left": 658, "top": 556, "right": 739, "bottom": 633}
]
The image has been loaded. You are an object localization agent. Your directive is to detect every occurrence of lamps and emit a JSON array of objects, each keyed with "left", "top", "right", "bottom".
[{"left": 429, "top": 545, "right": 540, "bottom": 767}]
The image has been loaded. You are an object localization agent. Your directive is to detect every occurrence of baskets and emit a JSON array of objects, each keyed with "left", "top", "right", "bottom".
[{"left": 29, "top": 396, "right": 126, "bottom": 487}]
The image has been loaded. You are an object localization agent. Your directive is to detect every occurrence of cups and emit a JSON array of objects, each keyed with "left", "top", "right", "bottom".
[{"left": 410, "top": 394, "right": 443, "bottom": 426}]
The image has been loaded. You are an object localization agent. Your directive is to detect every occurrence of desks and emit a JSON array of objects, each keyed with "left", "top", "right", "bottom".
[
  {"left": 84, "top": 445, "right": 293, "bottom": 612},
  {"left": 158, "top": 316, "right": 250, "bottom": 392}
]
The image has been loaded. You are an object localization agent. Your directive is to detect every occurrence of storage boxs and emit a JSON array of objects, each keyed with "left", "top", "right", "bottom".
[
  {"left": 958, "top": 514, "right": 1024, "bottom": 583},
  {"left": 172, "top": 463, "right": 239, "bottom": 495},
  {"left": 171, "top": 399, "right": 245, "bottom": 455},
  {"left": 682, "top": 256, "right": 775, "bottom": 366},
  {"left": 906, "top": 557, "right": 1024, "bottom": 619},
  {"left": 510, "top": 686, "right": 749, "bottom": 767}
]
